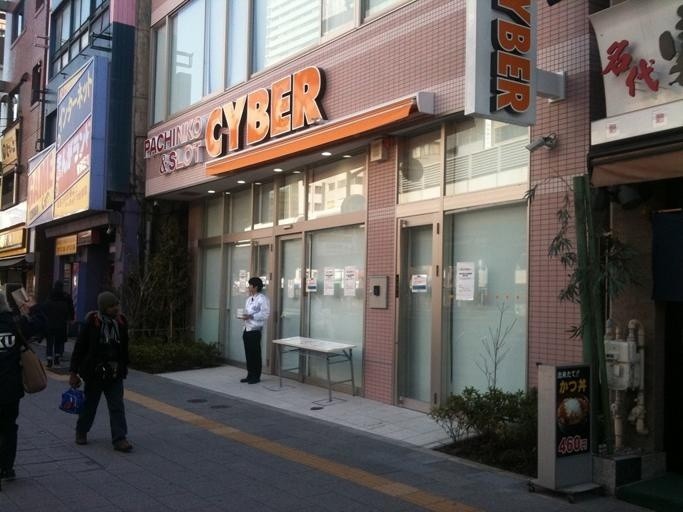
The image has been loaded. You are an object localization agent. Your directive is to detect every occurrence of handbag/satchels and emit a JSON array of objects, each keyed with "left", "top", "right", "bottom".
[
  {"left": 19, "top": 348, "right": 48, "bottom": 394},
  {"left": 59, "top": 386, "right": 83, "bottom": 414}
]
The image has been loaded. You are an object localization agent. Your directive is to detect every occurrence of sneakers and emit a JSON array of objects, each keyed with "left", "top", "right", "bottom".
[
  {"left": 240, "top": 378, "right": 260, "bottom": 384},
  {"left": 46, "top": 359, "right": 60, "bottom": 368},
  {"left": 113, "top": 438, "right": 132, "bottom": 451},
  {"left": 76, "top": 430, "right": 88, "bottom": 445}
]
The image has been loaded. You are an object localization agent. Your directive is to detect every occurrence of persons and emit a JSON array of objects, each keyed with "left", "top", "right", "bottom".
[
  {"left": 0, "top": 290, "right": 48, "bottom": 482},
  {"left": 43, "top": 281, "right": 75, "bottom": 368},
  {"left": 240, "top": 277, "right": 271, "bottom": 385},
  {"left": 68, "top": 291, "right": 133, "bottom": 451}
]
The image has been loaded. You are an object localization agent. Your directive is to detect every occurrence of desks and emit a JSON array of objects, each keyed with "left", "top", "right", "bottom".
[{"left": 272, "top": 335, "right": 357, "bottom": 403}]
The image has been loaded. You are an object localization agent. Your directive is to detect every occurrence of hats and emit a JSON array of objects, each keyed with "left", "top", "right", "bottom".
[{"left": 98, "top": 291, "right": 121, "bottom": 315}]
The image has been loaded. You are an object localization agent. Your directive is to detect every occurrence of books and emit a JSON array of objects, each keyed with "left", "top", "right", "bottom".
[{"left": 10, "top": 287, "right": 30, "bottom": 308}]
